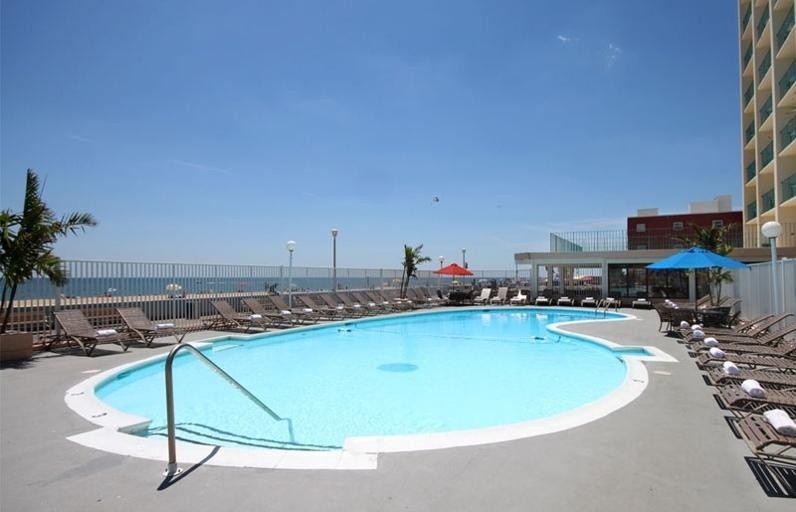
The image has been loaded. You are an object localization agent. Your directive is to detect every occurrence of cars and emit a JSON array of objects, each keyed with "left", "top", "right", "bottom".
[{"left": 475, "top": 268, "right": 601, "bottom": 287}]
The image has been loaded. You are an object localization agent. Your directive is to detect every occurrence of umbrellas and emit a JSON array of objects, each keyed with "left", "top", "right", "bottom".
[
  {"left": 643, "top": 247, "right": 751, "bottom": 311},
  {"left": 166, "top": 284, "right": 181, "bottom": 291},
  {"left": 106, "top": 288, "right": 117, "bottom": 293},
  {"left": 432, "top": 263, "right": 474, "bottom": 292}
]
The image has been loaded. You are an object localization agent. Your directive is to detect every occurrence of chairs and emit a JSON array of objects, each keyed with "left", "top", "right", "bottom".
[
  {"left": 472, "top": 280, "right": 650, "bottom": 315},
  {"left": 333, "top": 287, "right": 445, "bottom": 319},
  {"left": 206, "top": 295, "right": 347, "bottom": 334},
  {"left": 52, "top": 307, "right": 192, "bottom": 358},
  {"left": 654, "top": 293, "right": 796, "bottom": 474}
]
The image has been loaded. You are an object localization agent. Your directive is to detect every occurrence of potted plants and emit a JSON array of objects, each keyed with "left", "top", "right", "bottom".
[{"left": 0, "top": 165, "right": 96, "bottom": 361}]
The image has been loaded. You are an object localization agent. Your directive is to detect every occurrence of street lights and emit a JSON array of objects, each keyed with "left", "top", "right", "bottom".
[
  {"left": 761, "top": 220, "right": 783, "bottom": 324},
  {"left": 330, "top": 228, "right": 340, "bottom": 289},
  {"left": 287, "top": 240, "right": 297, "bottom": 310},
  {"left": 461, "top": 246, "right": 468, "bottom": 286},
  {"left": 438, "top": 254, "right": 444, "bottom": 295}
]
